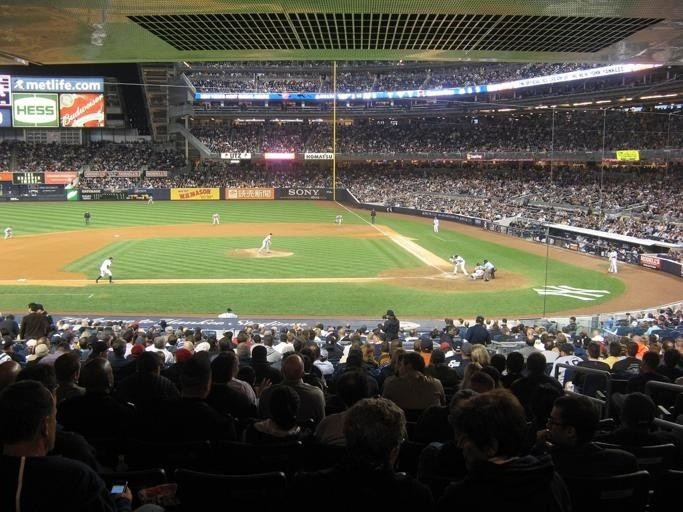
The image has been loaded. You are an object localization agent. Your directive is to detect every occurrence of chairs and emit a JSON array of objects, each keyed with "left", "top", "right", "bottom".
[{"left": 0, "top": 401, "right": 682, "bottom": 511}]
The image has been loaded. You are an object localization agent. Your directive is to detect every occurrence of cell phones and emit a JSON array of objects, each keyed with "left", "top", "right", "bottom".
[{"left": 110, "top": 482, "right": 128, "bottom": 494}]
